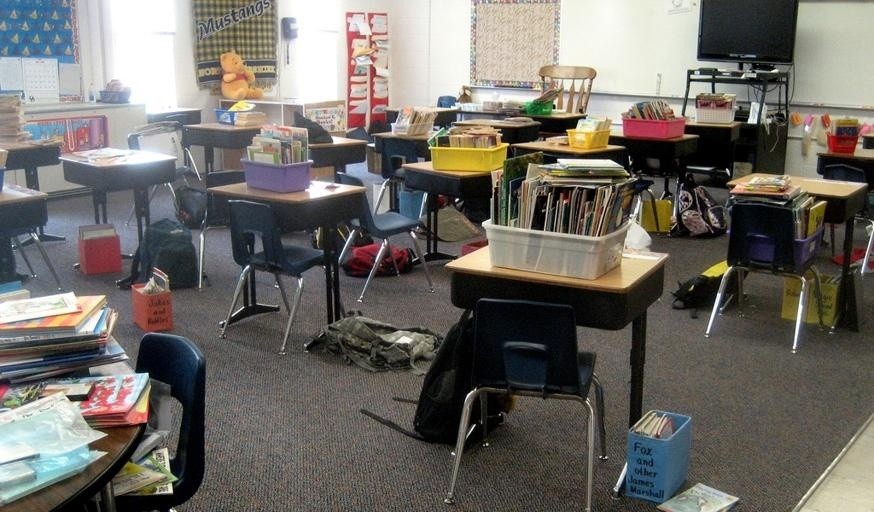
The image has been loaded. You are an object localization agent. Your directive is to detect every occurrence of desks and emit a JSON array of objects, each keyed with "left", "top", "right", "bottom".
[
  {"left": 370, "top": 132, "right": 436, "bottom": 213},
  {"left": 444, "top": 244, "right": 670, "bottom": 500},
  {"left": 511, "top": 141, "right": 627, "bottom": 165},
  {"left": 816, "top": 148, "right": 873, "bottom": 192},
  {"left": 718, "top": 173, "right": 868, "bottom": 335},
  {"left": 207, "top": 180, "right": 368, "bottom": 354},
  {"left": 530, "top": 114, "right": 588, "bottom": 134},
  {"left": 384, "top": 105, "right": 461, "bottom": 130},
  {"left": 686, "top": 120, "right": 742, "bottom": 190},
  {"left": 1, "top": 361, "right": 147, "bottom": 512},
  {"left": 59, "top": 148, "right": 179, "bottom": 289},
  {"left": 308, "top": 135, "right": 369, "bottom": 183},
  {"left": 184, "top": 122, "right": 262, "bottom": 174},
  {"left": 0, "top": 183, "right": 49, "bottom": 283},
  {"left": 610, "top": 132, "right": 700, "bottom": 200},
  {"left": 402, "top": 161, "right": 491, "bottom": 266},
  {"left": 1, "top": 139, "right": 67, "bottom": 250},
  {"left": 451, "top": 118, "right": 542, "bottom": 145}
]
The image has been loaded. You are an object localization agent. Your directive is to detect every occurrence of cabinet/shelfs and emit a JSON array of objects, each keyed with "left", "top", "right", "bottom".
[
  {"left": 682, "top": 66, "right": 791, "bottom": 177},
  {"left": 5, "top": 96, "right": 151, "bottom": 201}
]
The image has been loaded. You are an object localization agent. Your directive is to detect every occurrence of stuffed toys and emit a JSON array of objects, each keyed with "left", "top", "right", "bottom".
[{"left": 220, "top": 49, "right": 264, "bottom": 99}]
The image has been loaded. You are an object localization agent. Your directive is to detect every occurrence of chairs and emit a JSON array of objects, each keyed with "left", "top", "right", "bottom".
[
  {"left": 703, "top": 202, "right": 824, "bottom": 355},
  {"left": 79, "top": 332, "right": 207, "bottom": 512},
  {"left": 332, "top": 171, "right": 436, "bottom": 304},
  {"left": 442, "top": 298, "right": 610, "bottom": 510},
  {"left": 125, "top": 124, "right": 193, "bottom": 227},
  {"left": 218, "top": 199, "right": 348, "bottom": 355},
  {"left": 539, "top": 65, "right": 597, "bottom": 113}
]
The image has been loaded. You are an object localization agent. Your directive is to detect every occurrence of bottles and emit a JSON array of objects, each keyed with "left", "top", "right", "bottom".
[{"left": 89, "top": 81, "right": 96, "bottom": 104}]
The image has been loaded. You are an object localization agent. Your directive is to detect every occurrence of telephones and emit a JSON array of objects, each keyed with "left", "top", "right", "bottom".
[{"left": 281, "top": 16, "right": 299, "bottom": 40}]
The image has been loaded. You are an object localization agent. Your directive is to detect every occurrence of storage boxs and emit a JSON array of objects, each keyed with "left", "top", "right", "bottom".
[
  {"left": 781, "top": 271, "right": 817, "bottom": 321},
  {"left": 0, "top": 167, "right": 8, "bottom": 193},
  {"left": 390, "top": 122, "right": 435, "bottom": 136},
  {"left": 428, "top": 143, "right": 510, "bottom": 171},
  {"left": 77, "top": 234, "right": 122, "bottom": 275},
  {"left": 566, "top": 128, "right": 612, "bottom": 150},
  {"left": 400, "top": 190, "right": 426, "bottom": 219},
  {"left": 240, "top": 157, "right": 314, "bottom": 193},
  {"left": 132, "top": 281, "right": 174, "bottom": 334},
  {"left": 622, "top": 118, "right": 687, "bottom": 139},
  {"left": 523, "top": 101, "right": 554, "bottom": 114},
  {"left": 483, "top": 219, "right": 633, "bottom": 282},
  {"left": 825, "top": 131, "right": 859, "bottom": 154},
  {"left": 695, "top": 105, "right": 736, "bottom": 124},
  {"left": 639, "top": 200, "right": 672, "bottom": 233},
  {"left": 215, "top": 108, "right": 251, "bottom": 125},
  {"left": 624, "top": 409, "right": 691, "bottom": 503},
  {"left": 726, "top": 227, "right": 825, "bottom": 268}
]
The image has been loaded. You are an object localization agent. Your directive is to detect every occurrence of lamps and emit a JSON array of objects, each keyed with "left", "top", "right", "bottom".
[{"left": 806, "top": 275, "right": 842, "bottom": 327}]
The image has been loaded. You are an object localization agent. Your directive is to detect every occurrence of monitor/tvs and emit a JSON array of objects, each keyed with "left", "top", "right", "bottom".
[{"left": 696, "top": 1, "right": 799, "bottom": 66}]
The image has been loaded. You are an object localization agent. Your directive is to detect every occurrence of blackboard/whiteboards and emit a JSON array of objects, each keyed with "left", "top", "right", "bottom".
[{"left": 469, "top": 0, "right": 870, "bottom": 111}]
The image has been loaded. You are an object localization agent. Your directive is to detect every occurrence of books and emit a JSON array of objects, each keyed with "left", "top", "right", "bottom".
[
  {"left": 843, "top": 269, "right": 864, "bottom": 330},
  {"left": 0, "top": 148, "right": 9, "bottom": 169},
  {"left": 630, "top": 409, "right": 674, "bottom": 439},
  {"left": 79, "top": 223, "right": 116, "bottom": 239},
  {"left": 696, "top": 93, "right": 736, "bottom": 109},
  {"left": 0, "top": 280, "right": 128, "bottom": 384},
  {"left": 657, "top": 482, "right": 739, "bottom": 512},
  {"left": 826, "top": 275, "right": 843, "bottom": 286},
  {"left": 455, "top": 101, "right": 520, "bottom": 111},
  {"left": 831, "top": 120, "right": 859, "bottom": 135},
  {"left": 525, "top": 87, "right": 563, "bottom": 103},
  {"left": 490, "top": 152, "right": 654, "bottom": 279},
  {"left": 348, "top": 14, "right": 390, "bottom": 114},
  {"left": 575, "top": 113, "right": 612, "bottom": 132},
  {"left": 428, "top": 125, "right": 502, "bottom": 148},
  {"left": 0, "top": 371, "right": 152, "bottom": 429},
  {"left": 623, "top": 100, "right": 675, "bottom": 120},
  {"left": 394, "top": 105, "right": 437, "bottom": 124},
  {"left": 493, "top": 116, "right": 535, "bottom": 125},
  {"left": 87, "top": 430, "right": 179, "bottom": 502},
  {"left": 142, "top": 266, "right": 168, "bottom": 294},
  {"left": 0, "top": 93, "right": 32, "bottom": 144},
  {"left": 245, "top": 125, "right": 309, "bottom": 165},
  {"left": 729, "top": 174, "right": 828, "bottom": 240},
  {"left": 220, "top": 100, "right": 267, "bottom": 126},
  {"left": 73, "top": 145, "right": 133, "bottom": 160}
]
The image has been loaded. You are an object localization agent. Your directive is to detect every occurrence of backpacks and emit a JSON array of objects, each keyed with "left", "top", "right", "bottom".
[
  {"left": 320, "top": 316, "right": 444, "bottom": 373},
  {"left": 672, "top": 257, "right": 750, "bottom": 319},
  {"left": 344, "top": 242, "right": 415, "bottom": 277},
  {"left": 312, "top": 224, "right": 372, "bottom": 259},
  {"left": 675, "top": 172, "right": 726, "bottom": 238},
  {"left": 415, "top": 308, "right": 513, "bottom": 444},
  {"left": 171, "top": 186, "right": 227, "bottom": 228},
  {"left": 130, "top": 220, "right": 200, "bottom": 289}
]
